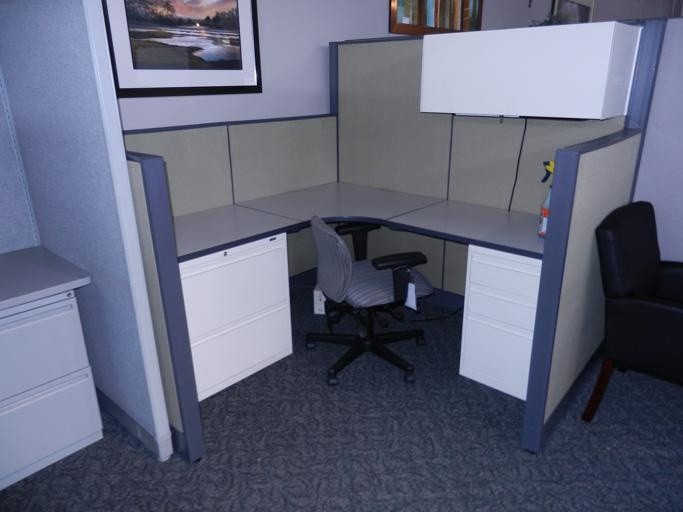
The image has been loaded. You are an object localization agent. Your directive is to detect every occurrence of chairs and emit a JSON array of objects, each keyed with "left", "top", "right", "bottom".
[
  {"left": 309, "top": 218, "right": 433, "bottom": 385},
  {"left": 579, "top": 196, "right": 682, "bottom": 422}
]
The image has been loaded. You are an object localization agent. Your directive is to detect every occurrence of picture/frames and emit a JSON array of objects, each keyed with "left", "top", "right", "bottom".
[
  {"left": 387, "top": 2, "right": 483, "bottom": 36},
  {"left": 101, "top": 1, "right": 260, "bottom": 95}
]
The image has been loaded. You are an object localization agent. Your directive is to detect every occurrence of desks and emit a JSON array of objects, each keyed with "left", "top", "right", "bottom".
[{"left": 176, "top": 182, "right": 548, "bottom": 324}]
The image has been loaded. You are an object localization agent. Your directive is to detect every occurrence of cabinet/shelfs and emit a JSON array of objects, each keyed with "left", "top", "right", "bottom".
[
  {"left": 418, "top": 20, "right": 647, "bottom": 124},
  {"left": 178, "top": 231, "right": 293, "bottom": 407},
  {"left": 458, "top": 243, "right": 542, "bottom": 403},
  {"left": 1, "top": 242, "right": 106, "bottom": 491}
]
